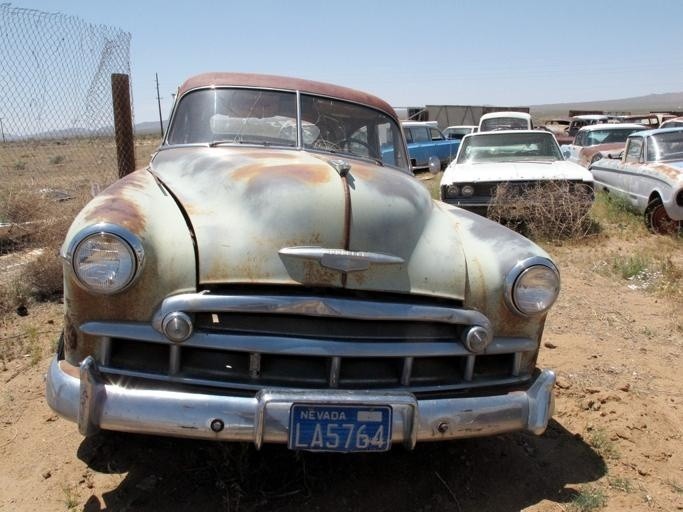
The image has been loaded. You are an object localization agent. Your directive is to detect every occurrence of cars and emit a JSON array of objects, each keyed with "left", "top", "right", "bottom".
[
  {"left": 379, "top": 119, "right": 462, "bottom": 174},
  {"left": 541, "top": 109, "right": 682, "bottom": 169},
  {"left": 438, "top": 130, "right": 594, "bottom": 224},
  {"left": 586, "top": 128, "right": 683, "bottom": 238},
  {"left": 42, "top": 69, "right": 561, "bottom": 458}
]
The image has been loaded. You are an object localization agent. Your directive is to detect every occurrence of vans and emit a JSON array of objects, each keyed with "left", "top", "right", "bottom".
[
  {"left": 443, "top": 124, "right": 480, "bottom": 142},
  {"left": 471, "top": 110, "right": 534, "bottom": 137}
]
